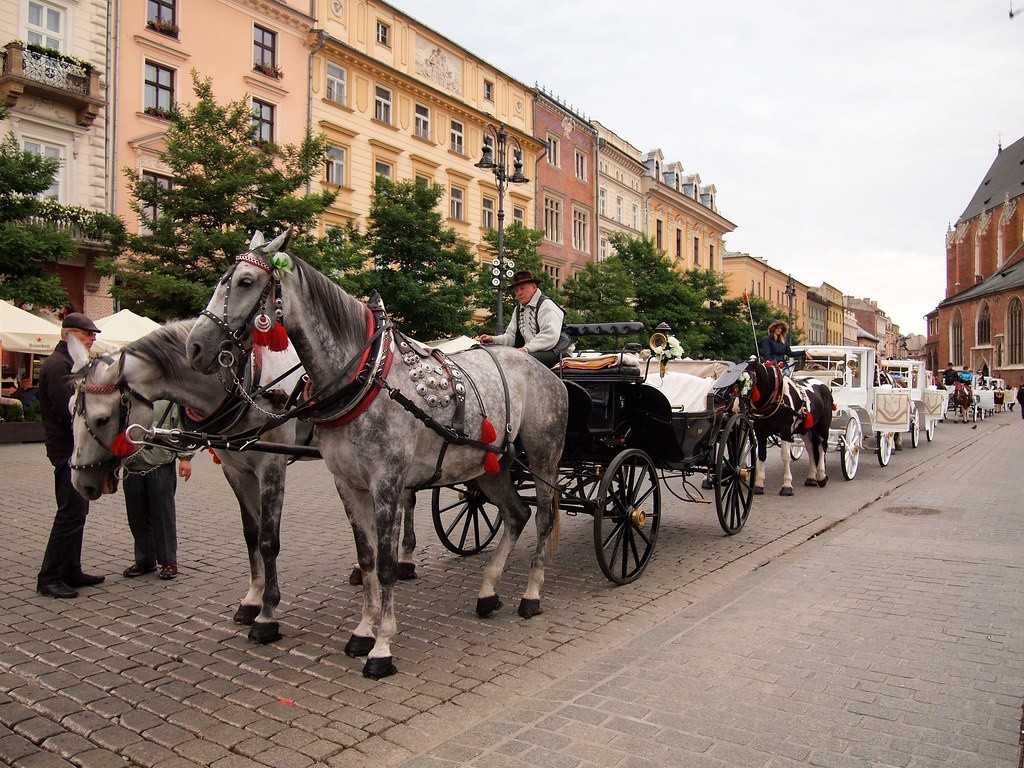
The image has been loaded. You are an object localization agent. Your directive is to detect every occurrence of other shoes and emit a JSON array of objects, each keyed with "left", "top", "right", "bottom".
[
  {"left": 36, "top": 578, "right": 79, "bottom": 598},
  {"left": 64, "top": 570, "right": 106, "bottom": 588},
  {"left": 123, "top": 561, "right": 158, "bottom": 576},
  {"left": 159, "top": 560, "right": 178, "bottom": 579}
]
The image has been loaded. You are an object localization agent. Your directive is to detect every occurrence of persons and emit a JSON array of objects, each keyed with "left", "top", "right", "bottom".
[
  {"left": 957, "top": 366, "right": 973, "bottom": 386},
  {"left": 1016, "top": 383, "right": 1024, "bottom": 419},
  {"left": 479, "top": 271, "right": 576, "bottom": 370},
  {"left": 122, "top": 400, "right": 196, "bottom": 580},
  {"left": 758, "top": 319, "right": 813, "bottom": 377},
  {"left": 35, "top": 313, "right": 104, "bottom": 598},
  {"left": 942, "top": 363, "right": 958, "bottom": 386},
  {"left": 10, "top": 373, "right": 35, "bottom": 400}
]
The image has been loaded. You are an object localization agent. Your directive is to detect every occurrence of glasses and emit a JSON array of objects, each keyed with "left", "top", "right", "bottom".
[{"left": 69, "top": 330, "right": 97, "bottom": 337}]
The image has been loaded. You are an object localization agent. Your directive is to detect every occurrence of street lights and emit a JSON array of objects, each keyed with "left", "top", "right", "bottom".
[
  {"left": 473, "top": 122, "right": 530, "bottom": 334},
  {"left": 782, "top": 274, "right": 796, "bottom": 345}
]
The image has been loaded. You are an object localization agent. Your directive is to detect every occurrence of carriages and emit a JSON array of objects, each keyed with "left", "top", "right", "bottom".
[
  {"left": 61, "top": 227, "right": 759, "bottom": 679},
  {"left": 878, "top": 357, "right": 944, "bottom": 449},
  {"left": 737, "top": 345, "right": 881, "bottom": 482},
  {"left": 939, "top": 367, "right": 1016, "bottom": 423}
]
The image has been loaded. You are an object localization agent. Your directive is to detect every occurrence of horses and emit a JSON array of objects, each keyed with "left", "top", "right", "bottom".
[
  {"left": 803, "top": 354, "right": 907, "bottom": 455},
  {"left": 953, "top": 380, "right": 972, "bottom": 424},
  {"left": 67, "top": 318, "right": 419, "bottom": 642},
  {"left": 702, "top": 361, "right": 833, "bottom": 497},
  {"left": 185, "top": 223, "right": 569, "bottom": 680}
]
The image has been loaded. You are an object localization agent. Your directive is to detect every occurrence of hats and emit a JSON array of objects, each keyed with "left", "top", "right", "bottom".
[
  {"left": 508, "top": 271, "right": 541, "bottom": 288},
  {"left": 62, "top": 313, "right": 101, "bottom": 333},
  {"left": 947, "top": 362, "right": 953, "bottom": 366},
  {"left": 21, "top": 372, "right": 31, "bottom": 380}
]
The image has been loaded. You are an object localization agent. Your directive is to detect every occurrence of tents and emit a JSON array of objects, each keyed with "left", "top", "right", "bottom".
[{"left": 1, "top": 300, "right": 162, "bottom": 382}]
